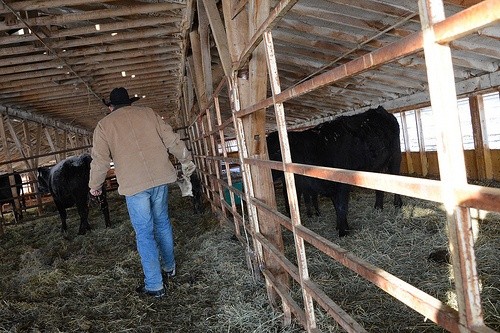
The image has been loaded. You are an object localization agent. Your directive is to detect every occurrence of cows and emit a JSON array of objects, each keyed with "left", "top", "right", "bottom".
[
  {"left": 37, "top": 154, "right": 111, "bottom": 235},
  {"left": 261, "top": 103, "right": 405, "bottom": 238},
  {"left": 0, "top": 171, "right": 26, "bottom": 226}
]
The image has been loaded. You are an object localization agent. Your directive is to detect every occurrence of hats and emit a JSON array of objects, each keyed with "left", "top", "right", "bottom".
[{"left": 101, "top": 87, "right": 140, "bottom": 107}]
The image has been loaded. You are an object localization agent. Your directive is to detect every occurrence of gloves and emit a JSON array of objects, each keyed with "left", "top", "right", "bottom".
[{"left": 182, "top": 162, "right": 196, "bottom": 178}]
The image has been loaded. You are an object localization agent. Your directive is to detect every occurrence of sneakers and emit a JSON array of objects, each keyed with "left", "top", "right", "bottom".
[
  {"left": 166, "top": 270, "right": 176, "bottom": 277},
  {"left": 136, "top": 286, "right": 166, "bottom": 296}
]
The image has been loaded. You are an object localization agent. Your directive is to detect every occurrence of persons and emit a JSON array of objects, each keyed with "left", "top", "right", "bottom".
[{"left": 87, "top": 86, "right": 196, "bottom": 297}]
